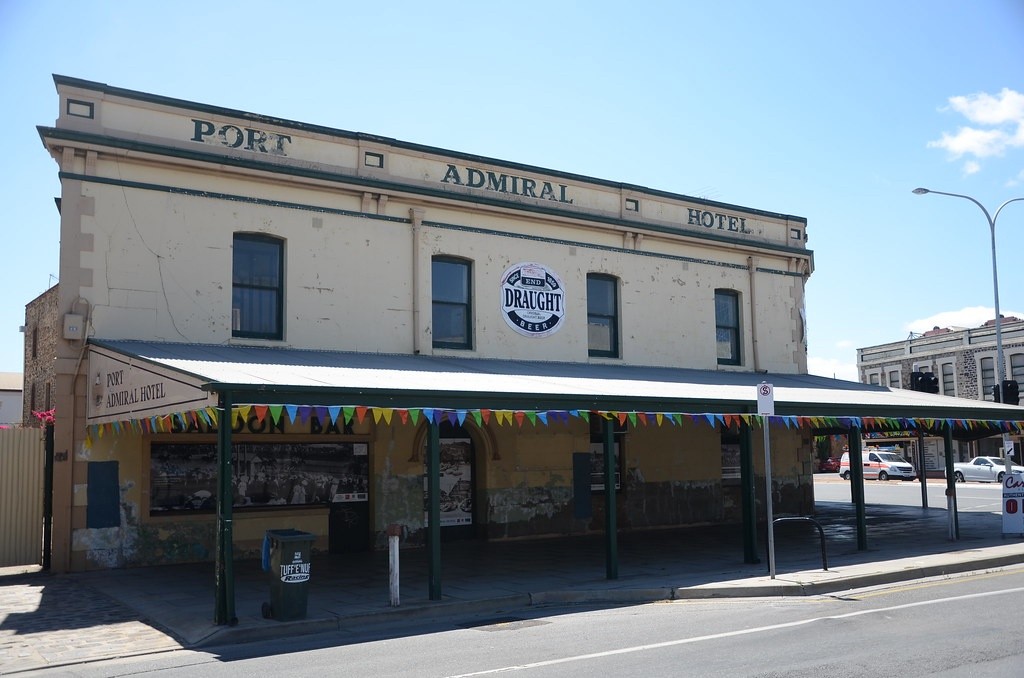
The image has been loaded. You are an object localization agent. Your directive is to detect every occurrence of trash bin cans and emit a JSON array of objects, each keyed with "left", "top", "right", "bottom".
[{"left": 261, "top": 528, "right": 316, "bottom": 621}]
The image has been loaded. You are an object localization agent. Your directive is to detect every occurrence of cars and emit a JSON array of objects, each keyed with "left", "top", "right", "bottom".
[{"left": 944, "top": 456, "right": 1024, "bottom": 483}]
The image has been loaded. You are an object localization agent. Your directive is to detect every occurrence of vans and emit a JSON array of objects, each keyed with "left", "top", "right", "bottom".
[{"left": 839, "top": 451, "right": 917, "bottom": 481}]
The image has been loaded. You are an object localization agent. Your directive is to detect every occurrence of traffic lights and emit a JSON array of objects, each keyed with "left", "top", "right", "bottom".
[
  {"left": 1003, "top": 380, "right": 1020, "bottom": 405},
  {"left": 909, "top": 372, "right": 939, "bottom": 392},
  {"left": 992, "top": 384, "right": 1000, "bottom": 403}
]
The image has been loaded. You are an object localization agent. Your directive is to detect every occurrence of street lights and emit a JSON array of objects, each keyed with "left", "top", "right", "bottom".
[{"left": 911, "top": 188, "right": 1024, "bottom": 475}]
